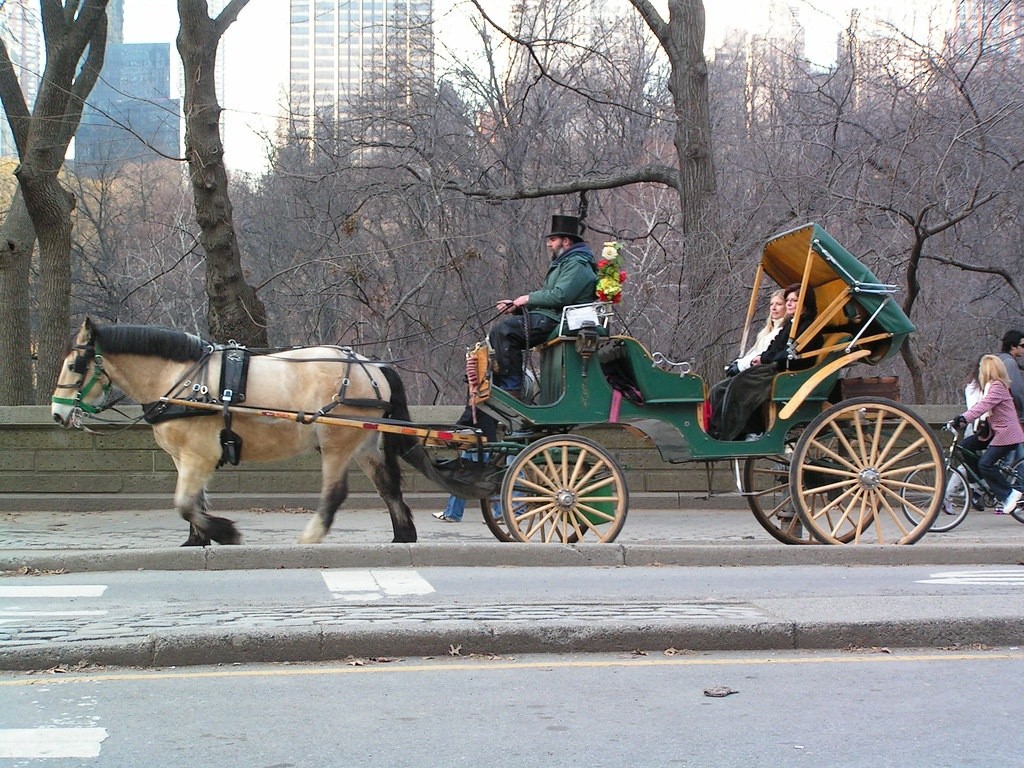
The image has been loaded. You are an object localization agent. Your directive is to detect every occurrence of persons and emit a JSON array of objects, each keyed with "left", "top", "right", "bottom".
[
  {"left": 946, "top": 354, "right": 1024, "bottom": 514},
  {"left": 997, "top": 328, "right": 1024, "bottom": 514},
  {"left": 706, "top": 287, "right": 793, "bottom": 439},
  {"left": 486, "top": 213, "right": 602, "bottom": 402},
  {"left": 721, "top": 284, "right": 826, "bottom": 442},
  {"left": 431, "top": 373, "right": 533, "bottom": 528},
  {"left": 940, "top": 351, "right": 994, "bottom": 514}
]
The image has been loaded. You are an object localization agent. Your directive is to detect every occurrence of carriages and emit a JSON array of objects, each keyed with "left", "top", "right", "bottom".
[{"left": 51, "top": 221, "right": 947, "bottom": 547}]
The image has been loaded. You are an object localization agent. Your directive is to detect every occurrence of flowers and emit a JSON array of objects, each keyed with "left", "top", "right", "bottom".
[{"left": 595, "top": 240, "right": 628, "bottom": 304}]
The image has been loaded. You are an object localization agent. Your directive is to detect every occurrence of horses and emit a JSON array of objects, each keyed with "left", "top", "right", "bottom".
[{"left": 51, "top": 314, "right": 425, "bottom": 545}]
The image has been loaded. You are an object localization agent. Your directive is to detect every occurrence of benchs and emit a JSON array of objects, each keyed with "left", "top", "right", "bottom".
[
  {"left": 766, "top": 332, "right": 852, "bottom": 431},
  {"left": 610, "top": 334, "right": 709, "bottom": 404},
  {"left": 533, "top": 323, "right": 609, "bottom": 353}
]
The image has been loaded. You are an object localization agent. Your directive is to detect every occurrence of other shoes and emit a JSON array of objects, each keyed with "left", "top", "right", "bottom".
[
  {"left": 483, "top": 519, "right": 505, "bottom": 524},
  {"left": 432, "top": 512, "right": 456, "bottom": 521},
  {"left": 995, "top": 508, "right": 1003, "bottom": 514},
  {"left": 507, "top": 390, "right": 522, "bottom": 402},
  {"left": 745, "top": 432, "right": 763, "bottom": 441},
  {"left": 972, "top": 498, "right": 984, "bottom": 511},
  {"left": 942, "top": 504, "right": 955, "bottom": 515}
]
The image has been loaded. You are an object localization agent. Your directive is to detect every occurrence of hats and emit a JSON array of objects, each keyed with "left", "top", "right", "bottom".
[{"left": 546, "top": 215, "right": 584, "bottom": 243}]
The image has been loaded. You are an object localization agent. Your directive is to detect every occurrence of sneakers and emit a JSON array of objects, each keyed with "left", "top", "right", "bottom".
[
  {"left": 969, "top": 479, "right": 989, "bottom": 488},
  {"left": 1003, "top": 489, "right": 1022, "bottom": 513}
]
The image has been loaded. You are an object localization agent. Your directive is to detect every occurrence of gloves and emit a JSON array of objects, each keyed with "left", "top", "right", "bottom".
[
  {"left": 726, "top": 364, "right": 738, "bottom": 378},
  {"left": 725, "top": 362, "right": 736, "bottom": 373},
  {"left": 954, "top": 417, "right": 965, "bottom": 428}
]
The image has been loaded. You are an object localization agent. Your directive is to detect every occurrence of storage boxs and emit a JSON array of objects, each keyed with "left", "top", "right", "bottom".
[{"left": 830, "top": 376, "right": 901, "bottom": 420}]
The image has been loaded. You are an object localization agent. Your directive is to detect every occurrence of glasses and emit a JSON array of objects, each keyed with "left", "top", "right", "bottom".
[{"left": 1019, "top": 344, "right": 1024, "bottom": 348}]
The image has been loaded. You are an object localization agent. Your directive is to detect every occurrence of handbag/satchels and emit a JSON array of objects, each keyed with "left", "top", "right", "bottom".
[{"left": 974, "top": 390, "right": 987, "bottom": 433}]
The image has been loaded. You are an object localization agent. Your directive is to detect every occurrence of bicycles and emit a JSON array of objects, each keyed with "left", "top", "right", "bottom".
[{"left": 899, "top": 416, "right": 1024, "bottom": 532}]
